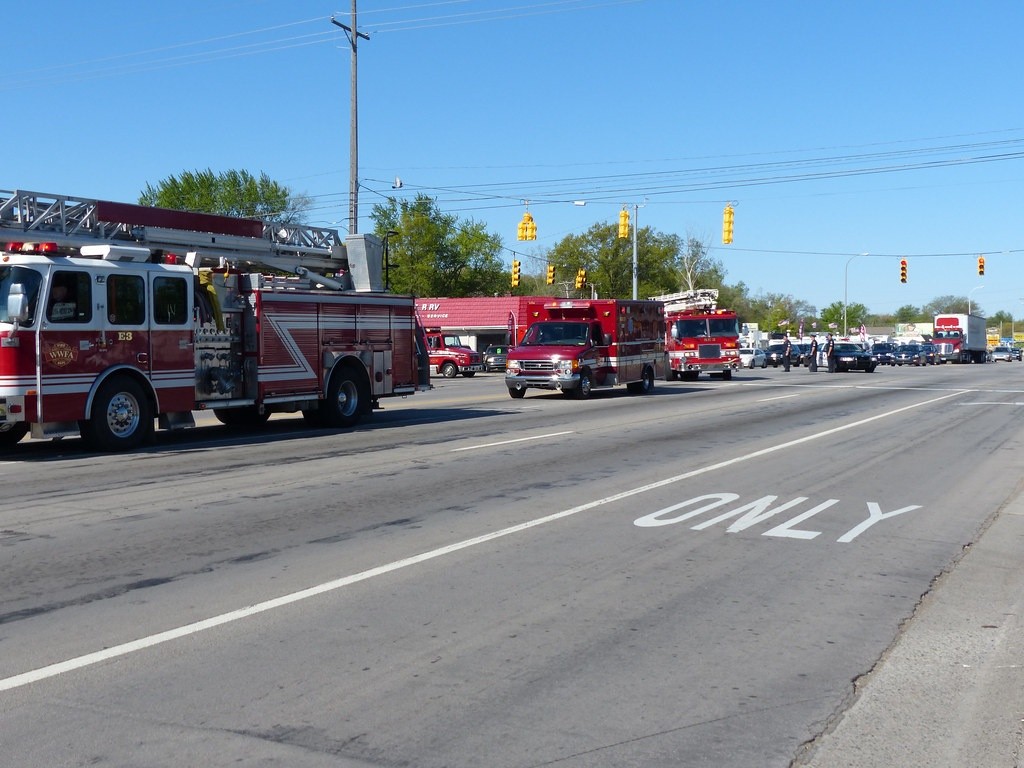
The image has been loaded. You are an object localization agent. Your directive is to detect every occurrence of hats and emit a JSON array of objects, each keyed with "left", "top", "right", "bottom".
[
  {"left": 783, "top": 334, "right": 788, "bottom": 337},
  {"left": 53, "top": 276, "right": 68, "bottom": 288},
  {"left": 811, "top": 334, "right": 816, "bottom": 337},
  {"left": 828, "top": 333, "right": 833, "bottom": 336}
]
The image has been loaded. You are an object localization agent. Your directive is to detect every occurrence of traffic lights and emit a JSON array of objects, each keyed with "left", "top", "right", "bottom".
[
  {"left": 978, "top": 258, "right": 985, "bottom": 276},
  {"left": 515, "top": 261, "right": 521, "bottom": 286},
  {"left": 900, "top": 259, "right": 907, "bottom": 284},
  {"left": 549, "top": 265, "right": 555, "bottom": 284},
  {"left": 578, "top": 270, "right": 587, "bottom": 289}
]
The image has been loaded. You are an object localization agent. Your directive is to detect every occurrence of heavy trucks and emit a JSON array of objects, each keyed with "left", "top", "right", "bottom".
[{"left": 932, "top": 313, "right": 987, "bottom": 365}]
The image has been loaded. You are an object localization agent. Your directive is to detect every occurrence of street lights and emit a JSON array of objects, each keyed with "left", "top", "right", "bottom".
[
  {"left": 968, "top": 285, "right": 984, "bottom": 315},
  {"left": 844, "top": 253, "right": 870, "bottom": 337}
]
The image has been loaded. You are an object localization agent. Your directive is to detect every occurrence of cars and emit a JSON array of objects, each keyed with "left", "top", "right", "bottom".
[
  {"left": 739, "top": 342, "right": 942, "bottom": 373},
  {"left": 988, "top": 347, "right": 1023, "bottom": 363},
  {"left": 483, "top": 343, "right": 510, "bottom": 373}
]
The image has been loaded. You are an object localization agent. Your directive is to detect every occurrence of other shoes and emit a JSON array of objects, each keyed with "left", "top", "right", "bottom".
[
  {"left": 782, "top": 369, "right": 790, "bottom": 372},
  {"left": 827, "top": 370, "right": 833, "bottom": 373},
  {"left": 810, "top": 369, "right": 817, "bottom": 372}
]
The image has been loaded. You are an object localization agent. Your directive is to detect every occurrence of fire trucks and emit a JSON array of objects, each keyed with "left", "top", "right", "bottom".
[
  {"left": 0, "top": 189, "right": 433, "bottom": 451},
  {"left": 505, "top": 299, "right": 667, "bottom": 398},
  {"left": 646, "top": 289, "right": 745, "bottom": 381},
  {"left": 424, "top": 327, "right": 483, "bottom": 378}
]
